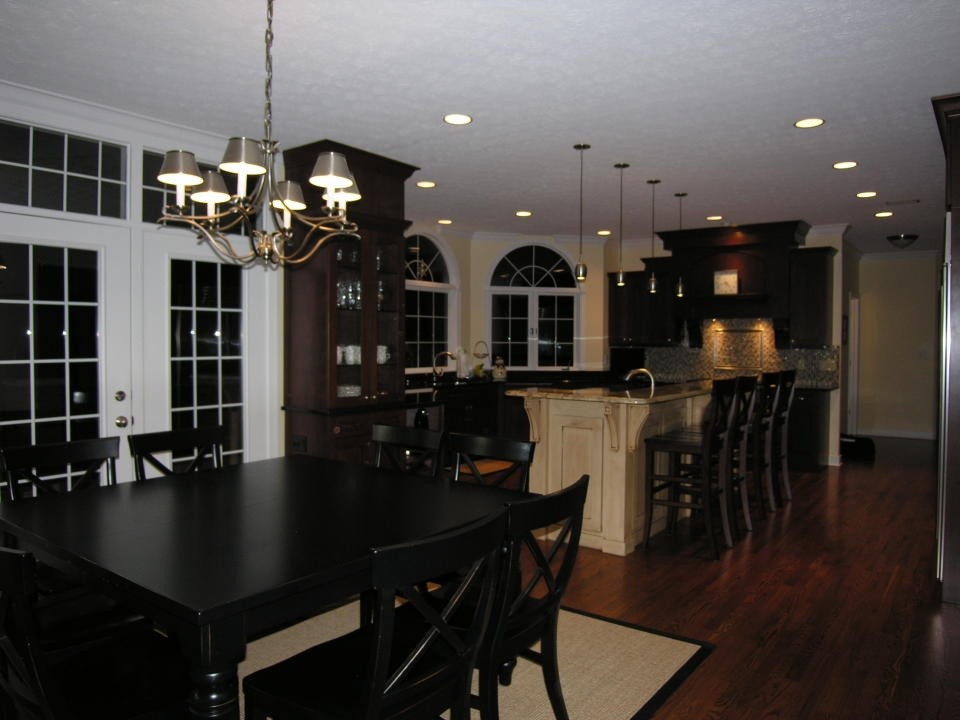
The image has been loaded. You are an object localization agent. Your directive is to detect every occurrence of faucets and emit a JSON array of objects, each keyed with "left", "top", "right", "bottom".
[
  {"left": 433, "top": 351, "right": 457, "bottom": 385},
  {"left": 624, "top": 367, "right": 655, "bottom": 396}
]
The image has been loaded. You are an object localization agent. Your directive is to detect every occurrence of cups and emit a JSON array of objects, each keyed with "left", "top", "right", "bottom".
[{"left": 337, "top": 344, "right": 391, "bottom": 366}]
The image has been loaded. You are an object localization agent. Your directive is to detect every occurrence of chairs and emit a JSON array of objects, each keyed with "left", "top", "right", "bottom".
[
  {"left": 0, "top": 435, "right": 194, "bottom": 720},
  {"left": 127, "top": 423, "right": 229, "bottom": 483},
  {"left": 241, "top": 504, "right": 510, "bottom": 720},
  {"left": 356, "top": 419, "right": 448, "bottom": 629},
  {"left": 370, "top": 430, "right": 536, "bottom": 629},
  {"left": 639, "top": 368, "right": 797, "bottom": 560},
  {"left": 372, "top": 474, "right": 590, "bottom": 720}
]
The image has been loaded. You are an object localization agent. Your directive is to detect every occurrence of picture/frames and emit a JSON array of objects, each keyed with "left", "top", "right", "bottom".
[{"left": 842, "top": 314, "right": 849, "bottom": 346}]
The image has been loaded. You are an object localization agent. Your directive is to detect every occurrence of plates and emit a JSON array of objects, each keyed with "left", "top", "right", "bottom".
[{"left": 337, "top": 384, "right": 360, "bottom": 397}]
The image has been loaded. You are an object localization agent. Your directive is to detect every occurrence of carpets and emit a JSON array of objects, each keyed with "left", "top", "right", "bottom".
[
  {"left": 238, "top": 579, "right": 720, "bottom": 720},
  {"left": 443, "top": 458, "right": 515, "bottom": 479}
]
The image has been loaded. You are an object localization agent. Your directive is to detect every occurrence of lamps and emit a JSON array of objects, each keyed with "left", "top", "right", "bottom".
[
  {"left": 573, "top": 143, "right": 592, "bottom": 282},
  {"left": 673, "top": 192, "right": 688, "bottom": 298},
  {"left": 886, "top": 233, "right": 920, "bottom": 249},
  {"left": 613, "top": 162, "right": 631, "bottom": 289},
  {"left": 645, "top": 178, "right": 662, "bottom": 295},
  {"left": 154, "top": 0, "right": 365, "bottom": 273}
]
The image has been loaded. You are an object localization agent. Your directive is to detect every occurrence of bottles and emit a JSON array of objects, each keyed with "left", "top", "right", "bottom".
[
  {"left": 337, "top": 242, "right": 384, "bottom": 312},
  {"left": 493, "top": 356, "right": 507, "bottom": 378}
]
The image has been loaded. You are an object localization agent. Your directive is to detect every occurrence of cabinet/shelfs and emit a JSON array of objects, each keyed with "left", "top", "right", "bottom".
[
  {"left": 789, "top": 246, "right": 840, "bottom": 349},
  {"left": 437, "top": 385, "right": 499, "bottom": 437},
  {"left": 639, "top": 255, "right": 703, "bottom": 348},
  {"left": 280, "top": 139, "right": 423, "bottom": 469},
  {"left": 607, "top": 270, "right": 645, "bottom": 347},
  {"left": 787, "top": 388, "right": 830, "bottom": 475},
  {"left": 498, "top": 384, "right": 552, "bottom": 442}
]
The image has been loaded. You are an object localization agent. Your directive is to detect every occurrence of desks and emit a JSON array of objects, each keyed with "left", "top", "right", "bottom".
[{"left": 0, "top": 453, "right": 545, "bottom": 720}]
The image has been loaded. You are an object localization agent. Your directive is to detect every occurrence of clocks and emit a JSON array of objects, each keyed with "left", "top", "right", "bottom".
[{"left": 713, "top": 268, "right": 739, "bottom": 296}]
